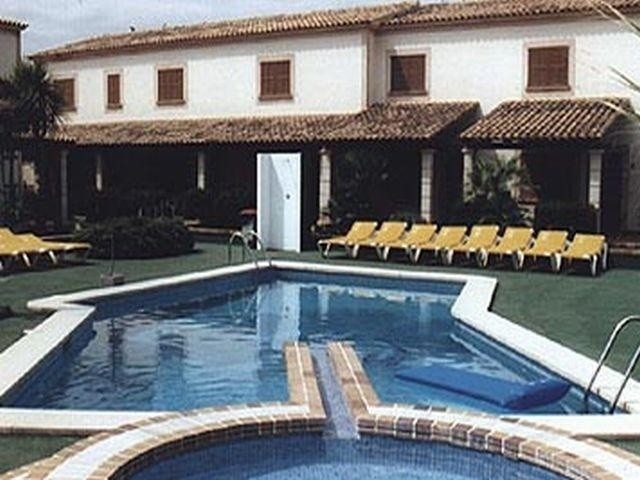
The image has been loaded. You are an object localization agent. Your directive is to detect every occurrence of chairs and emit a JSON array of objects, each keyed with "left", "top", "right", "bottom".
[
  {"left": 0, "top": 227, "right": 91, "bottom": 270},
  {"left": 318, "top": 221, "right": 608, "bottom": 277}
]
